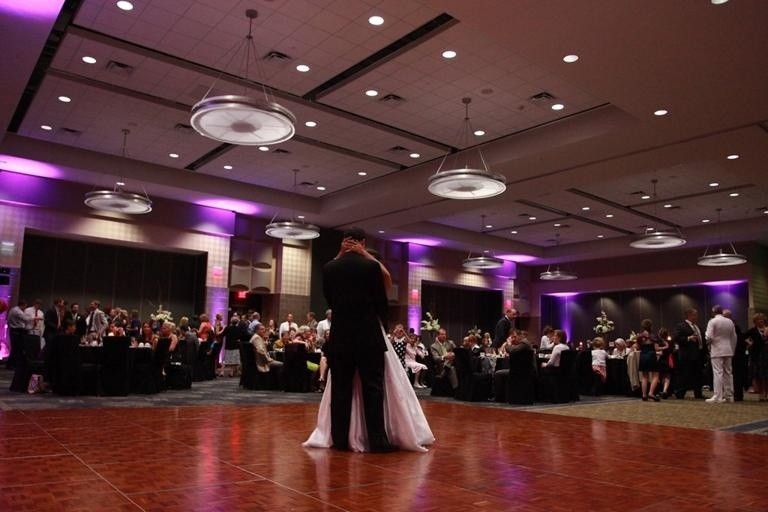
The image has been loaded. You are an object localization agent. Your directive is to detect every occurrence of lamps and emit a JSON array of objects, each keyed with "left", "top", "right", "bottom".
[
  {"left": 628, "top": 177, "right": 687, "bottom": 250},
  {"left": 695, "top": 208, "right": 747, "bottom": 269},
  {"left": 426, "top": 96, "right": 506, "bottom": 201},
  {"left": 189, "top": 9, "right": 296, "bottom": 150},
  {"left": 458, "top": 215, "right": 504, "bottom": 271},
  {"left": 80, "top": 126, "right": 154, "bottom": 217},
  {"left": 537, "top": 235, "right": 580, "bottom": 284},
  {"left": 262, "top": 168, "right": 321, "bottom": 245}
]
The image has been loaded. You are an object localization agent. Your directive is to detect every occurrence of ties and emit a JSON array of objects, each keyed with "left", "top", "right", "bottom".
[
  {"left": 88, "top": 312, "right": 94, "bottom": 330},
  {"left": 35, "top": 309, "right": 37, "bottom": 327}
]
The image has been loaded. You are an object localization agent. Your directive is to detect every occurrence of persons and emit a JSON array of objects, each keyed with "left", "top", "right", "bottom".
[
  {"left": 493, "top": 309, "right": 518, "bottom": 350},
  {"left": 389, "top": 324, "right": 632, "bottom": 389},
  {"left": 302, "top": 236, "right": 436, "bottom": 453},
  {"left": 322, "top": 227, "right": 392, "bottom": 452},
  {"left": 7, "top": 297, "right": 332, "bottom": 394},
  {"left": 637, "top": 304, "right": 768, "bottom": 401}
]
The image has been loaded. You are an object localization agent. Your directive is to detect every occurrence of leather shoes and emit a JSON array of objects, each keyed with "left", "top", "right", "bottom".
[
  {"left": 642, "top": 389, "right": 743, "bottom": 403},
  {"left": 372, "top": 445, "right": 400, "bottom": 452},
  {"left": 413, "top": 383, "right": 428, "bottom": 389}
]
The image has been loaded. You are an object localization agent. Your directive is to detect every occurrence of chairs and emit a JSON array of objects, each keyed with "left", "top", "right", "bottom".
[{"left": 17, "top": 327, "right": 642, "bottom": 404}]
